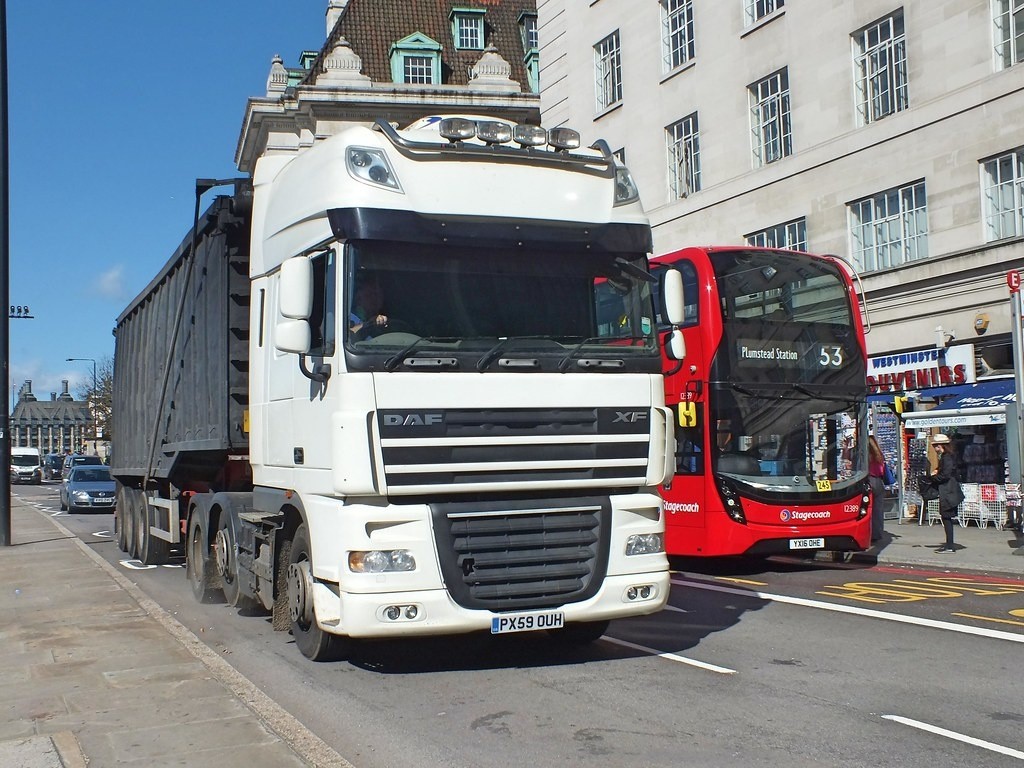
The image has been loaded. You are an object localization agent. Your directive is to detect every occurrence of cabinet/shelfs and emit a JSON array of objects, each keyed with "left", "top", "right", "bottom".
[{"left": 928, "top": 483, "right": 1007, "bottom": 532}]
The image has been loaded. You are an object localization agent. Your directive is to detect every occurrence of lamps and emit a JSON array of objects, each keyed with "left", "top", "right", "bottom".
[{"left": 934, "top": 326, "right": 956, "bottom": 348}]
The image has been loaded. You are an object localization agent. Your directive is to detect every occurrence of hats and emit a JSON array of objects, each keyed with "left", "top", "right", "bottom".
[{"left": 931, "top": 434, "right": 951, "bottom": 444}]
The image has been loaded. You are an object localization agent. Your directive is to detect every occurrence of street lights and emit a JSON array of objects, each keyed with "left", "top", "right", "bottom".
[{"left": 66, "top": 358, "right": 96, "bottom": 454}]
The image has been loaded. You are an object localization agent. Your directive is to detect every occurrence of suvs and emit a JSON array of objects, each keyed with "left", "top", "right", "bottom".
[
  {"left": 44, "top": 453, "right": 66, "bottom": 480},
  {"left": 61, "top": 455, "right": 105, "bottom": 481}
]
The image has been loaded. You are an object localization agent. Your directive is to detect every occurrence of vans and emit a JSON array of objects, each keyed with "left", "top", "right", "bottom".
[{"left": 10, "top": 447, "right": 44, "bottom": 485}]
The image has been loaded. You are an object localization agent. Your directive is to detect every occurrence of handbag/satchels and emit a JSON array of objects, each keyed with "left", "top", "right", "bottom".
[
  {"left": 918, "top": 476, "right": 939, "bottom": 501},
  {"left": 881, "top": 462, "right": 896, "bottom": 485}
]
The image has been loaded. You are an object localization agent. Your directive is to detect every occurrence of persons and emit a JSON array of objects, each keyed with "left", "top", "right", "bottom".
[
  {"left": 321, "top": 275, "right": 387, "bottom": 345},
  {"left": 842, "top": 435, "right": 885, "bottom": 541},
  {"left": 929, "top": 434, "right": 965, "bottom": 553}
]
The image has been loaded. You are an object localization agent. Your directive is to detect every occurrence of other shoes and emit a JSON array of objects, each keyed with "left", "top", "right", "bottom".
[{"left": 934, "top": 547, "right": 955, "bottom": 554}]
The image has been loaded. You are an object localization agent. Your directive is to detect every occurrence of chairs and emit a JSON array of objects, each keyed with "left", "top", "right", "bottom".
[
  {"left": 76, "top": 472, "right": 86, "bottom": 481},
  {"left": 716, "top": 457, "right": 762, "bottom": 476},
  {"left": 97, "top": 471, "right": 111, "bottom": 481}
]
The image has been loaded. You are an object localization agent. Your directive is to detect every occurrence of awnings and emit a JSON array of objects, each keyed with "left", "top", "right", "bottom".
[{"left": 901, "top": 379, "right": 1017, "bottom": 429}]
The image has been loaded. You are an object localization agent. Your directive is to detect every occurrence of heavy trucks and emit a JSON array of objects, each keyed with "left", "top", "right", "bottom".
[{"left": 110, "top": 112, "right": 687, "bottom": 663}]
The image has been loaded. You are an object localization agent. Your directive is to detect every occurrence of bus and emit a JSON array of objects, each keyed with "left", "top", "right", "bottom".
[{"left": 589, "top": 244, "right": 908, "bottom": 558}]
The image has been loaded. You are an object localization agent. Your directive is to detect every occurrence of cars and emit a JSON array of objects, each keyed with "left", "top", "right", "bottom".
[{"left": 60, "top": 465, "right": 118, "bottom": 514}]
